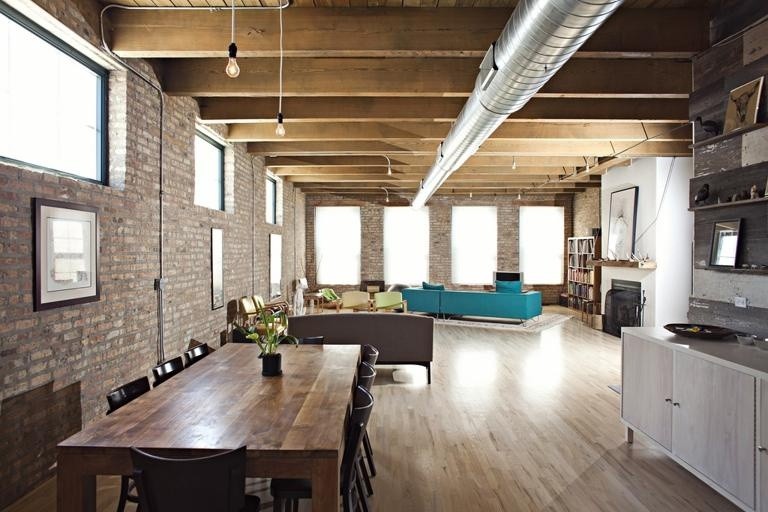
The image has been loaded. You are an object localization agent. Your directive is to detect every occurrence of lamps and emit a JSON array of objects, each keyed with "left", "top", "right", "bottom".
[
  {"left": 273, "top": 0, "right": 288, "bottom": 138},
  {"left": 225, "top": 1, "right": 242, "bottom": 78}
]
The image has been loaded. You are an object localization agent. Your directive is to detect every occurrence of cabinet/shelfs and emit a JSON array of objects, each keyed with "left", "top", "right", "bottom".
[
  {"left": 689, "top": 16, "right": 768, "bottom": 340},
  {"left": 621, "top": 334, "right": 757, "bottom": 511},
  {"left": 567, "top": 237, "right": 602, "bottom": 315}
]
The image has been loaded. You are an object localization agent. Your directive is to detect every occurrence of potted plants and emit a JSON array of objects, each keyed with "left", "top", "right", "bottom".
[{"left": 234, "top": 301, "right": 299, "bottom": 376}]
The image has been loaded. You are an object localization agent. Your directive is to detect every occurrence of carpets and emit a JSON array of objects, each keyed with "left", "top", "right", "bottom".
[{"left": 434, "top": 313, "right": 575, "bottom": 333}]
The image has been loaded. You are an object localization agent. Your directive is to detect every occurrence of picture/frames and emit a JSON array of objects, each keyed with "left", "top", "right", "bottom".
[
  {"left": 269, "top": 233, "right": 282, "bottom": 301},
  {"left": 723, "top": 76, "right": 764, "bottom": 133},
  {"left": 33, "top": 197, "right": 101, "bottom": 311},
  {"left": 210, "top": 228, "right": 225, "bottom": 311},
  {"left": 608, "top": 186, "right": 639, "bottom": 262}
]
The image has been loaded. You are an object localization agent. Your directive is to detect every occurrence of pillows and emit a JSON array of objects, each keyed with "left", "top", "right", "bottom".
[
  {"left": 423, "top": 281, "right": 445, "bottom": 290},
  {"left": 495, "top": 281, "right": 522, "bottom": 293}
]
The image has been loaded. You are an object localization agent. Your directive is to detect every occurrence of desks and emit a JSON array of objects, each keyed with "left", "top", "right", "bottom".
[
  {"left": 304, "top": 292, "right": 323, "bottom": 313},
  {"left": 56, "top": 341, "right": 361, "bottom": 512}
]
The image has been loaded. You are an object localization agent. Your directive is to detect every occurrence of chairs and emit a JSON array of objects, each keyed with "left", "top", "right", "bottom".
[
  {"left": 356, "top": 343, "right": 380, "bottom": 456},
  {"left": 349, "top": 361, "right": 377, "bottom": 497},
  {"left": 336, "top": 290, "right": 371, "bottom": 313},
  {"left": 152, "top": 357, "right": 185, "bottom": 384},
  {"left": 252, "top": 294, "right": 289, "bottom": 324},
  {"left": 278, "top": 332, "right": 325, "bottom": 346},
  {"left": 373, "top": 291, "right": 408, "bottom": 313},
  {"left": 240, "top": 296, "right": 282, "bottom": 335},
  {"left": 183, "top": 343, "right": 210, "bottom": 366},
  {"left": 106, "top": 375, "right": 151, "bottom": 511},
  {"left": 270, "top": 386, "right": 375, "bottom": 512},
  {"left": 129, "top": 445, "right": 262, "bottom": 512}
]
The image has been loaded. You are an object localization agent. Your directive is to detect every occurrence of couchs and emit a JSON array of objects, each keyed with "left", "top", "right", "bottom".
[
  {"left": 288, "top": 312, "right": 434, "bottom": 385},
  {"left": 402, "top": 289, "right": 542, "bottom": 327}
]
